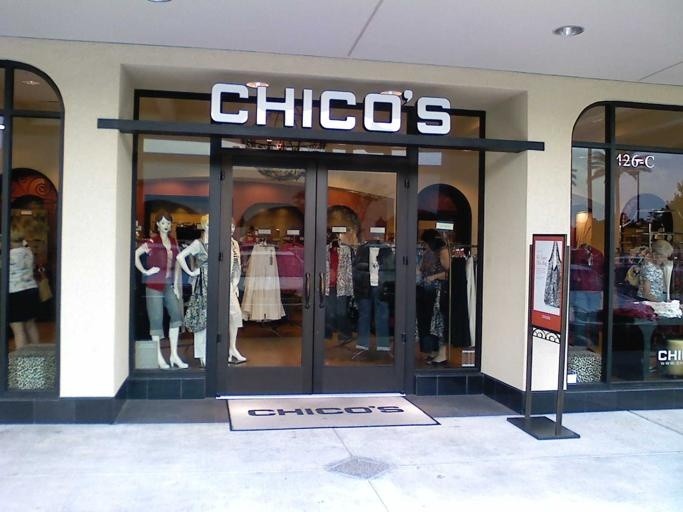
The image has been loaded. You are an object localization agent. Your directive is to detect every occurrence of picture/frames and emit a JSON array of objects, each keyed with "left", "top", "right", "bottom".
[{"left": 528, "top": 233, "right": 568, "bottom": 334}]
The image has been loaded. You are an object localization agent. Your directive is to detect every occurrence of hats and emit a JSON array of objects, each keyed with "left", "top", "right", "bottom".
[
  {"left": 653, "top": 240, "right": 673, "bottom": 257},
  {"left": 422, "top": 228, "right": 445, "bottom": 251}
]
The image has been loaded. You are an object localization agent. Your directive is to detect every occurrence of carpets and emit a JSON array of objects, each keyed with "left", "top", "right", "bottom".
[{"left": 225, "top": 395, "right": 441, "bottom": 431}]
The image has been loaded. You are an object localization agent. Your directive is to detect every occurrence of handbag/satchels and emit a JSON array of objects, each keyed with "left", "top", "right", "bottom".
[
  {"left": 185, "top": 294, "right": 207, "bottom": 333},
  {"left": 37, "top": 269, "right": 53, "bottom": 302},
  {"left": 625, "top": 265, "right": 641, "bottom": 286}
]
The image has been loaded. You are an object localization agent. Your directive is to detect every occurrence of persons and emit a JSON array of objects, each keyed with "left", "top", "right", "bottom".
[
  {"left": 227, "top": 217, "right": 246, "bottom": 365},
  {"left": 6, "top": 225, "right": 39, "bottom": 350},
  {"left": 633, "top": 240, "right": 673, "bottom": 304},
  {"left": 414, "top": 228, "right": 451, "bottom": 365},
  {"left": 175, "top": 214, "right": 208, "bottom": 368},
  {"left": 133, "top": 208, "right": 188, "bottom": 371}
]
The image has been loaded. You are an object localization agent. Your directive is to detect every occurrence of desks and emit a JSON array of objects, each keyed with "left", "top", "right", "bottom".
[{"left": 576, "top": 311, "right": 683, "bottom": 377}]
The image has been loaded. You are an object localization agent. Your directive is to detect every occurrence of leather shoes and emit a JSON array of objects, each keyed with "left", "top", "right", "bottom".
[{"left": 425, "top": 354, "right": 449, "bottom": 366}]
